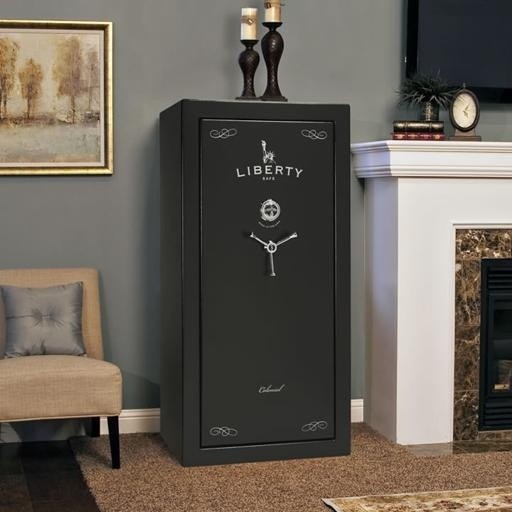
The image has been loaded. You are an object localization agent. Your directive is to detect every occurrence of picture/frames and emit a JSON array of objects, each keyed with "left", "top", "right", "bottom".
[{"left": 0, "top": 19, "right": 115, "bottom": 178}]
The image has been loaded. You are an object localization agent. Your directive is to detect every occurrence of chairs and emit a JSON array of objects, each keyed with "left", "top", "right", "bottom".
[{"left": 0, "top": 267, "right": 124, "bottom": 470}]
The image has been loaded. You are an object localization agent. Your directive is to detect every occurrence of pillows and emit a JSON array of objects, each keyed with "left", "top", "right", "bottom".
[{"left": 1, "top": 281, "right": 86, "bottom": 358}]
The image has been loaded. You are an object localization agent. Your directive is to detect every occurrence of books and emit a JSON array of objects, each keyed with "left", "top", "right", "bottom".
[
  {"left": 390, "top": 132, "right": 445, "bottom": 141},
  {"left": 392, "top": 120, "right": 444, "bottom": 133}
]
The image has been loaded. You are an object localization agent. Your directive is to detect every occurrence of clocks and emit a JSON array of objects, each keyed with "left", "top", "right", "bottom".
[{"left": 447, "top": 83, "right": 483, "bottom": 141}]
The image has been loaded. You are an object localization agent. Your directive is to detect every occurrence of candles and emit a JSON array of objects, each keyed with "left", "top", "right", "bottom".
[
  {"left": 265, "top": 0, "right": 281, "bottom": 21},
  {"left": 241, "top": 8, "right": 257, "bottom": 40}
]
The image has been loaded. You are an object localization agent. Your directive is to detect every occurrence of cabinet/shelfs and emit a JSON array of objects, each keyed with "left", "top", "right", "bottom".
[{"left": 161, "top": 99, "right": 349, "bottom": 467}]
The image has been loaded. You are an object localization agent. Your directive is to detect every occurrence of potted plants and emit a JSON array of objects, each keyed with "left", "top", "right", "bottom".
[{"left": 394, "top": 69, "right": 463, "bottom": 122}]
